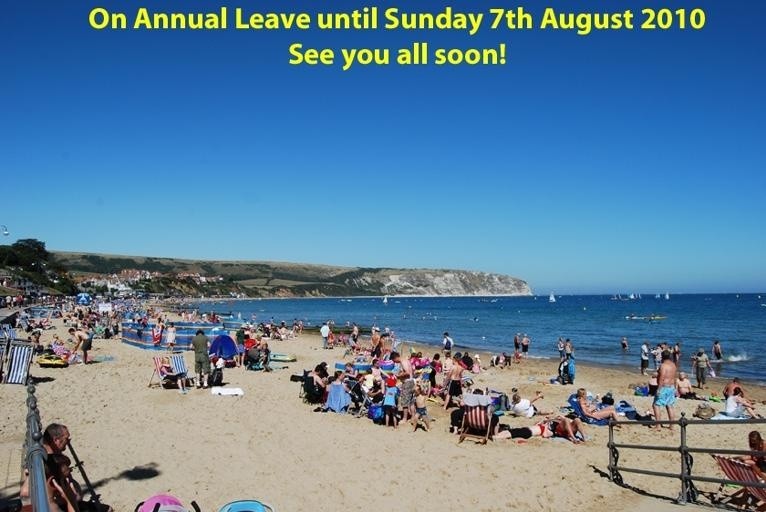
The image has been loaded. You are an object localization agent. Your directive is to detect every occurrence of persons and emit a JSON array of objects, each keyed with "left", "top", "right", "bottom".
[
  {"left": 744, "top": 454, "right": 766, "bottom": 479},
  {"left": 38, "top": 455, "right": 114, "bottom": 511},
  {"left": 736, "top": 431, "right": 766, "bottom": 461},
  {"left": 18, "top": 422, "right": 71, "bottom": 511}
]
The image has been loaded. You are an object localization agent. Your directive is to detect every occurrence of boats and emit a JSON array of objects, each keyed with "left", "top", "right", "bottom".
[{"left": 624, "top": 315, "right": 669, "bottom": 321}]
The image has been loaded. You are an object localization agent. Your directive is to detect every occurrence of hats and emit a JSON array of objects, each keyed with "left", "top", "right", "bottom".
[
  {"left": 698, "top": 348, "right": 704, "bottom": 353},
  {"left": 387, "top": 378, "right": 396, "bottom": 387},
  {"left": 474, "top": 354, "right": 480, "bottom": 359}
]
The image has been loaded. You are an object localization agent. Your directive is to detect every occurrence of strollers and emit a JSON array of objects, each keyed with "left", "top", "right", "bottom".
[{"left": 130, "top": 495, "right": 278, "bottom": 512}]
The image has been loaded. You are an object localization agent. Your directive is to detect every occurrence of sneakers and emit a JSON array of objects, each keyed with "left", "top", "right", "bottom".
[
  {"left": 178, "top": 387, "right": 189, "bottom": 394},
  {"left": 196, "top": 381, "right": 201, "bottom": 387},
  {"left": 203, "top": 381, "right": 208, "bottom": 387}
]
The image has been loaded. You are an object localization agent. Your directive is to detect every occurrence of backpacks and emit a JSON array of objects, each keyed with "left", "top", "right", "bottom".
[
  {"left": 209, "top": 368, "right": 223, "bottom": 386},
  {"left": 497, "top": 394, "right": 511, "bottom": 411},
  {"left": 472, "top": 363, "right": 480, "bottom": 374}
]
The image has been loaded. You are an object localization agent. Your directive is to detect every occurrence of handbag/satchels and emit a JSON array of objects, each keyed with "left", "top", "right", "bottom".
[
  {"left": 367, "top": 404, "right": 385, "bottom": 425},
  {"left": 635, "top": 386, "right": 648, "bottom": 397},
  {"left": 692, "top": 404, "right": 716, "bottom": 421}
]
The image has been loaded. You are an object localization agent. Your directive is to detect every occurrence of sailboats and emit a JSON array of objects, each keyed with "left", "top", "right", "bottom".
[
  {"left": 548, "top": 291, "right": 557, "bottom": 303},
  {"left": 607, "top": 287, "right": 672, "bottom": 302}
]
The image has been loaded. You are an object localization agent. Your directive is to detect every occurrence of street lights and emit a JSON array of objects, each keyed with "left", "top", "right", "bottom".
[
  {"left": 56, "top": 275, "right": 66, "bottom": 307},
  {"left": 31, "top": 257, "right": 47, "bottom": 310},
  {"left": 0, "top": 224, "right": 10, "bottom": 238}
]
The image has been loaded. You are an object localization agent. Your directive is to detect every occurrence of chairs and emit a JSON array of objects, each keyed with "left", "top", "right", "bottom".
[
  {"left": 0, "top": 300, "right": 766, "bottom": 446},
  {"left": 711, "top": 453, "right": 766, "bottom": 511}
]
